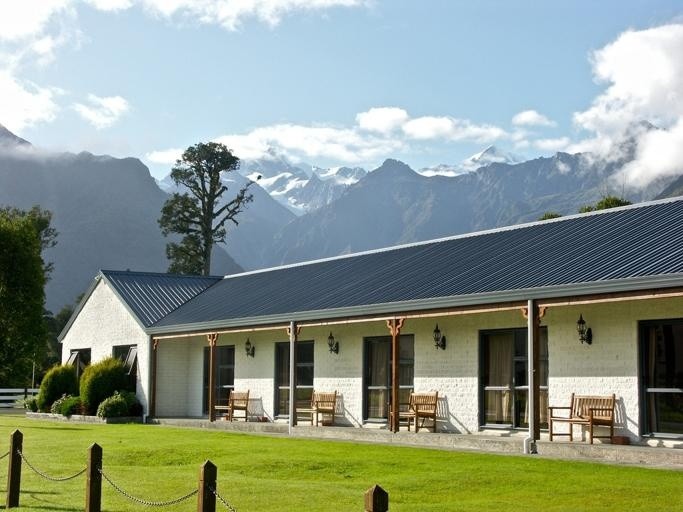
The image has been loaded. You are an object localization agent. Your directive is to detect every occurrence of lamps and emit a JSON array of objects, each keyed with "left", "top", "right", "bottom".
[
  {"left": 327, "top": 330, "right": 338, "bottom": 354},
  {"left": 244, "top": 337, "right": 255, "bottom": 358},
  {"left": 575, "top": 313, "right": 592, "bottom": 344},
  {"left": 432, "top": 322, "right": 445, "bottom": 350}
]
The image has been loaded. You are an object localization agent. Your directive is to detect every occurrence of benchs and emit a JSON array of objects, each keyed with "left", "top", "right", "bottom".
[
  {"left": 547, "top": 392, "right": 615, "bottom": 445},
  {"left": 214, "top": 389, "right": 249, "bottom": 421},
  {"left": 389, "top": 390, "right": 438, "bottom": 433},
  {"left": 295, "top": 390, "right": 336, "bottom": 427}
]
